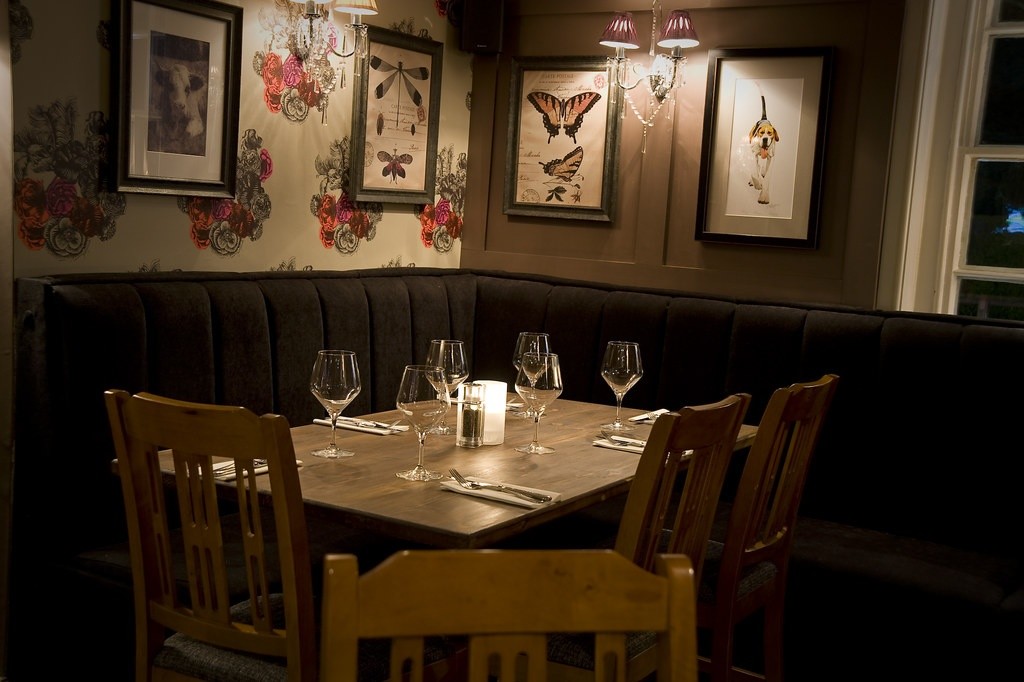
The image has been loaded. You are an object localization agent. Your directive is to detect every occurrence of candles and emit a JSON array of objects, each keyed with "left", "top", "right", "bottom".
[{"left": 466, "top": 378, "right": 508, "bottom": 444}]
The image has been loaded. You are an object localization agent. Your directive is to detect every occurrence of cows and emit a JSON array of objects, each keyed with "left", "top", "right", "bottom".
[{"left": 156, "top": 64, "right": 204, "bottom": 140}]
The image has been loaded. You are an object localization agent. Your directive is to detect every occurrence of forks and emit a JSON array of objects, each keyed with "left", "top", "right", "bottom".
[
  {"left": 600, "top": 430, "right": 645, "bottom": 449},
  {"left": 448, "top": 467, "right": 544, "bottom": 505},
  {"left": 646, "top": 412, "right": 660, "bottom": 419},
  {"left": 336, "top": 415, "right": 402, "bottom": 428}
]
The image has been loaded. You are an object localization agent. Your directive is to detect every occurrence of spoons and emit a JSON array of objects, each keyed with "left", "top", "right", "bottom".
[
  {"left": 325, "top": 417, "right": 377, "bottom": 428},
  {"left": 213, "top": 458, "right": 266, "bottom": 478}
]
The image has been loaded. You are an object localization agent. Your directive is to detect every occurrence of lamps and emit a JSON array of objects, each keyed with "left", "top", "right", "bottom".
[
  {"left": 290, "top": 0, "right": 380, "bottom": 131},
  {"left": 599, "top": 2, "right": 700, "bottom": 154}
]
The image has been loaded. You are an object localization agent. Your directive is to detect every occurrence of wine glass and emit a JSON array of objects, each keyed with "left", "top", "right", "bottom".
[
  {"left": 395, "top": 365, "right": 451, "bottom": 481},
  {"left": 514, "top": 352, "right": 563, "bottom": 454},
  {"left": 600, "top": 341, "right": 643, "bottom": 432},
  {"left": 310, "top": 350, "right": 361, "bottom": 459},
  {"left": 424, "top": 340, "right": 469, "bottom": 436},
  {"left": 512, "top": 332, "right": 552, "bottom": 418}
]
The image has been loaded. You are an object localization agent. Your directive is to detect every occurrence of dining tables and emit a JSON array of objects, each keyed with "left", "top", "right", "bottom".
[{"left": 106, "top": 385, "right": 766, "bottom": 679}]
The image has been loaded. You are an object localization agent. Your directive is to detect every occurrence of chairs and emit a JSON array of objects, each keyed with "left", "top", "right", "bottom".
[
  {"left": 98, "top": 387, "right": 447, "bottom": 682},
  {"left": 582, "top": 371, "right": 839, "bottom": 682},
  {"left": 514, "top": 387, "right": 749, "bottom": 680},
  {"left": 321, "top": 544, "right": 702, "bottom": 681}
]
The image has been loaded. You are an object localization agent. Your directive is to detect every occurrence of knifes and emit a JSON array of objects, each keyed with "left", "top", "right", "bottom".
[
  {"left": 445, "top": 475, "right": 552, "bottom": 500},
  {"left": 390, "top": 427, "right": 403, "bottom": 433},
  {"left": 595, "top": 435, "right": 646, "bottom": 447},
  {"left": 635, "top": 411, "right": 671, "bottom": 422}
]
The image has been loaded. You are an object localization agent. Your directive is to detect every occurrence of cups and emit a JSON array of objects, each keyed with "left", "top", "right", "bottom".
[
  {"left": 456, "top": 383, "right": 486, "bottom": 448},
  {"left": 471, "top": 380, "right": 507, "bottom": 445}
]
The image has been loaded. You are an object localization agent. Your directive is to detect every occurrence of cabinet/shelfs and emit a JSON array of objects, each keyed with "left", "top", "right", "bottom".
[{"left": 12, "top": 264, "right": 1022, "bottom": 682}]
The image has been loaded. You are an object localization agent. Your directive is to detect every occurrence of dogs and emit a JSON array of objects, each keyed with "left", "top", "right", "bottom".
[{"left": 747, "top": 95, "right": 779, "bottom": 204}]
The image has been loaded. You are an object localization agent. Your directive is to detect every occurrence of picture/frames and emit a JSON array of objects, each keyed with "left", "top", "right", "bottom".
[
  {"left": 689, "top": 44, "right": 836, "bottom": 252},
  {"left": 509, "top": 53, "right": 627, "bottom": 224},
  {"left": 346, "top": 23, "right": 446, "bottom": 206},
  {"left": 111, "top": 0, "right": 247, "bottom": 201}
]
